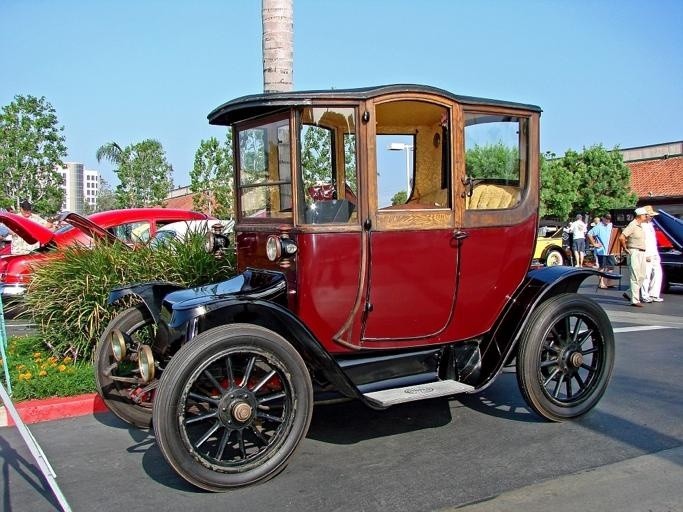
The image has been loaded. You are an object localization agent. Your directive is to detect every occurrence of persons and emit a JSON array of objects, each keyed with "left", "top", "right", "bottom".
[
  {"left": 587, "top": 216, "right": 601, "bottom": 268},
  {"left": 6, "top": 201, "right": 61, "bottom": 257},
  {"left": 566, "top": 220, "right": 576, "bottom": 267},
  {"left": 639, "top": 204, "right": 665, "bottom": 303},
  {"left": 0, "top": 208, "right": 14, "bottom": 240},
  {"left": 617, "top": 207, "right": 649, "bottom": 308},
  {"left": 588, "top": 212, "right": 619, "bottom": 289},
  {"left": 562, "top": 213, "right": 588, "bottom": 268}
]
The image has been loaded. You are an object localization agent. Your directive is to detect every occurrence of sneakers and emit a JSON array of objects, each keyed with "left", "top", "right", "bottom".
[
  {"left": 574, "top": 263, "right": 599, "bottom": 270},
  {"left": 623, "top": 292, "right": 666, "bottom": 308}
]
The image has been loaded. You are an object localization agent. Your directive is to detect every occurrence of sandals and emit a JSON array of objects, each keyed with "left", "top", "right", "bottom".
[{"left": 598, "top": 282, "right": 619, "bottom": 290}]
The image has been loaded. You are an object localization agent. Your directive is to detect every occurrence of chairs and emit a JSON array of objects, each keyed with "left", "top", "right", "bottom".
[{"left": 465, "top": 180, "right": 521, "bottom": 209}]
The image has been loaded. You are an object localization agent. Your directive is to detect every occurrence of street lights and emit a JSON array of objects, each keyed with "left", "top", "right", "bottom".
[{"left": 387, "top": 143, "right": 413, "bottom": 200}]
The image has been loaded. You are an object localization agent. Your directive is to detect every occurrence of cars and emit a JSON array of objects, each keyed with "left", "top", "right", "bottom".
[
  {"left": 652, "top": 209, "right": 683, "bottom": 294},
  {"left": 1, "top": 208, "right": 265, "bottom": 297},
  {"left": 531, "top": 226, "right": 568, "bottom": 268},
  {"left": 92, "top": 84, "right": 623, "bottom": 493}
]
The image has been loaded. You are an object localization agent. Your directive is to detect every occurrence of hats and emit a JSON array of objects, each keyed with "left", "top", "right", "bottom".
[
  {"left": 22, "top": 200, "right": 32, "bottom": 212},
  {"left": 575, "top": 213, "right": 582, "bottom": 219},
  {"left": 634, "top": 204, "right": 660, "bottom": 219},
  {"left": 602, "top": 213, "right": 613, "bottom": 219}
]
